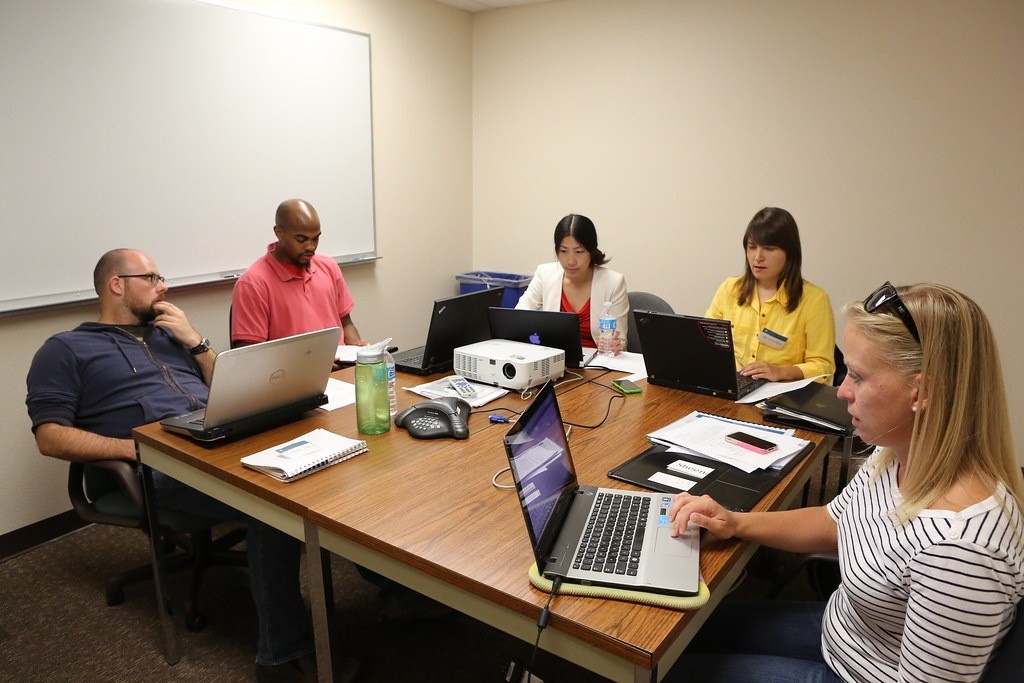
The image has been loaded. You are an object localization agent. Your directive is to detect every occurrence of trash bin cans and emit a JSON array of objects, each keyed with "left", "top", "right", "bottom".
[{"left": 456, "top": 271, "right": 533, "bottom": 308}]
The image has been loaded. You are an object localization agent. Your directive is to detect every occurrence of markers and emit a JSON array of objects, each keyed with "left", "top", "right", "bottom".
[{"left": 224, "top": 274, "right": 241, "bottom": 278}]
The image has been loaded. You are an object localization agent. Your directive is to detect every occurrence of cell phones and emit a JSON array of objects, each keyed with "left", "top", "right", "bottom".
[
  {"left": 725, "top": 431, "right": 778, "bottom": 454},
  {"left": 612, "top": 380, "right": 643, "bottom": 393}
]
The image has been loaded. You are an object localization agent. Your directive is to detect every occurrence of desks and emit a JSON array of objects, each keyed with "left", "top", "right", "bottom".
[{"left": 133, "top": 358, "right": 855, "bottom": 683}]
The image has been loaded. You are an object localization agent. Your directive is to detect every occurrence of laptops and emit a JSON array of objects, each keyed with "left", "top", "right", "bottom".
[
  {"left": 159, "top": 326, "right": 342, "bottom": 439},
  {"left": 633, "top": 309, "right": 768, "bottom": 400},
  {"left": 391, "top": 286, "right": 505, "bottom": 376},
  {"left": 504, "top": 378, "right": 701, "bottom": 596},
  {"left": 488, "top": 307, "right": 599, "bottom": 368}
]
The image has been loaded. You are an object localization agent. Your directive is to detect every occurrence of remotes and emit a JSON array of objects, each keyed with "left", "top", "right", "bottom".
[{"left": 450, "top": 375, "right": 477, "bottom": 398}]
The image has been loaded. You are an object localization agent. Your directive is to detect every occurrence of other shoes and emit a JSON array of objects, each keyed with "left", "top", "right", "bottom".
[{"left": 256, "top": 658, "right": 307, "bottom": 683}]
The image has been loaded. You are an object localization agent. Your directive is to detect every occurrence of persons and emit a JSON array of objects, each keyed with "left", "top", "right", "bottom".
[
  {"left": 230, "top": 198, "right": 367, "bottom": 371},
  {"left": 512, "top": 213, "right": 630, "bottom": 357},
  {"left": 663, "top": 279, "right": 1024, "bottom": 683},
  {"left": 703, "top": 207, "right": 835, "bottom": 384},
  {"left": 25, "top": 248, "right": 359, "bottom": 683}
]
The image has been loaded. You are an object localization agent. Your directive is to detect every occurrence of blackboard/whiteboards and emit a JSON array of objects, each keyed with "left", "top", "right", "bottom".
[{"left": 0, "top": 0, "right": 383, "bottom": 318}]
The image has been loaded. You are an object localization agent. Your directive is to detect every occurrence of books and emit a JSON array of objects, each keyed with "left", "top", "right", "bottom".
[{"left": 240, "top": 427, "right": 369, "bottom": 484}]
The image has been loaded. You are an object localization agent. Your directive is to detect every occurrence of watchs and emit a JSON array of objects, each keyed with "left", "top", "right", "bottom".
[{"left": 188, "top": 337, "right": 210, "bottom": 356}]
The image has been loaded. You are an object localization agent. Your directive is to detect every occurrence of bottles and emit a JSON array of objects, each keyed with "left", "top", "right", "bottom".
[
  {"left": 598, "top": 302, "right": 616, "bottom": 358},
  {"left": 355, "top": 338, "right": 397, "bottom": 435}
]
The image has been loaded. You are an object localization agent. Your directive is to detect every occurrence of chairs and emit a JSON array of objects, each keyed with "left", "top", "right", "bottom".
[
  {"left": 64, "top": 442, "right": 255, "bottom": 629},
  {"left": 625, "top": 290, "right": 677, "bottom": 356}
]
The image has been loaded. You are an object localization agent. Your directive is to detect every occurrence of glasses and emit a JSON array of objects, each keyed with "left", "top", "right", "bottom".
[
  {"left": 862, "top": 280, "right": 921, "bottom": 347},
  {"left": 115, "top": 273, "right": 164, "bottom": 288}
]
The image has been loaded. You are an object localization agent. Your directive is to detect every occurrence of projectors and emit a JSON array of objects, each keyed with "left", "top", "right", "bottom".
[{"left": 453, "top": 338, "right": 565, "bottom": 391}]
